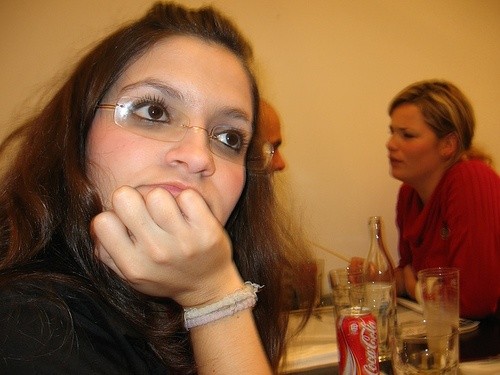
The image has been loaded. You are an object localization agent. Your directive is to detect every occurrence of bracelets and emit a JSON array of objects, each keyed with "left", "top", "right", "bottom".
[{"left": 184, "top": 283, "right": 257, "bottom": 331}]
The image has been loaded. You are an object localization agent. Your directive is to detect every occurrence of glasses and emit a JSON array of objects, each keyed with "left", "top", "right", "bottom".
[{"left": 96, "top": 96, "right": 274, "bottom": 170}]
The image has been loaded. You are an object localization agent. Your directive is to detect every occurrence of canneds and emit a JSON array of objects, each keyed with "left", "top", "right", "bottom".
[{"left": 336, "top": 299, "right": 380, "bottom": 375}]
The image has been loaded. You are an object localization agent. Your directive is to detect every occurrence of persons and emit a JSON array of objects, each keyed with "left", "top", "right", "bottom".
[
  {"left": 349, "top": 79, "right": 500, "bottom": 375},
  {"left": 255, "top": 100, "right": 286, "bottom": 173},
  {"left": 0, "top": 0, "right": 315, "bottom": 375}
]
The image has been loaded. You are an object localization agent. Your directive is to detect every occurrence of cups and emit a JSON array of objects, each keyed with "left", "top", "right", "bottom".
[
  {"left": 389, "top": 319, "right": 460, "bottom": 375},
  {"left": 417, "top": 267, "right": 459, "bottom": 375},
  {"left": 348, "top": 284, "right": 397, "bottom": 362},
  {"left": 329, "top": 268, "right": 366, "bottom": 312},
  {"left": 296, "top": 258, "right": 325, "bottom": 309}
]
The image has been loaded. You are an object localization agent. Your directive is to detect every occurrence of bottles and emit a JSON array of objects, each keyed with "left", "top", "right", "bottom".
[{"left": 361, "top": 215, "right": 398, "bottom": 306}]
